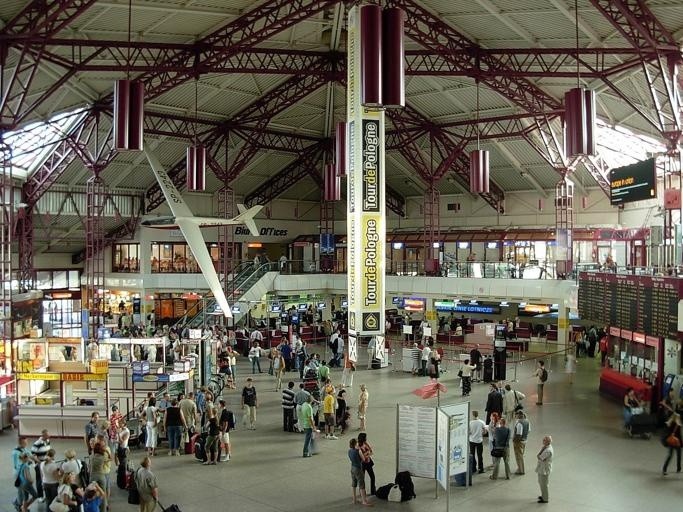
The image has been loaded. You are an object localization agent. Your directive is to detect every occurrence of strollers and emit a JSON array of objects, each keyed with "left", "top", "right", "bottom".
[{"left": 626, "top": 404, "right": 653, "bottom": 440}]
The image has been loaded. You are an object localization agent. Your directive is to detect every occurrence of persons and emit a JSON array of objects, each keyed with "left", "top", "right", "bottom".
[
  {"left": 268, "top": 304, "right": 356, "bottom": 457},
  {"left": 417, "top": 342, "right": 441, "bottom": 378},
  {"left": 535, "top": 360, "right": 547, "bottom": 405},
  {"left": 606, "top": 255, "right": 615, "bottom": 270},
  {"left": 507, "top": 317, "right": 516, "bottom": 340},
  {"left": 137, "top": 386, "right": 231, "bottom": 465},
  {"left": 575, "top": 326, "right": 609, "bottom": 368},
  {"left": 201, "top": 324, "right": 264, "bottom": 388},
  {"left": 14, "top": 406, "right": 159, "bottom": 512},
  {"left": 253, "top": 252, "right": 270, "bottom": 271},
  {"left": 468, "top": 253, "right": 476, "bottom": 262},
  {"left": 104, "top": 299, "right": 181, "bottom": 365},
  {"left": 535, "top": 436, "right": 553, "bottom": 503},
  {"left": 468, "top": 384, "right": 529, "bottom": 480},
  {"left": 459, "top": 343, "right": 482, "bottom": 396},
  {"left": 241, "top": 378, "right": 257, "bottom": 430},
  {"left": 623, "top": 388, "right": 683, "bottom": 475},
  {"left": 627, "top": 262, "right": 678, "bottom": 277},
  {"left": 279, "top": 253, "right": 287, "bottom": 270},
  {"left": 348, "top": 433, "right": 376, "bottom": 506},
  {"left": 437, "top": 312, "right": 462, "bottom": 335}
]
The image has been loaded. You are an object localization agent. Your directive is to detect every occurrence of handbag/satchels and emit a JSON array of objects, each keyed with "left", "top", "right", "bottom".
[
  {"left": 48, "top": 485, "right": 70, "bottom": 512},
  {"left": 14, "top": 463, "right": 24, "bottom": 488},
  {"left": 218, "top": 356, "right": 229, "bottom": 369},
  {"left": 484, "top": 358, "right": 493, "bottom": 367},
  {"left": 387, "top": 484, "right": 402, "bottom": 502},
  {"left": 457, "top": 365, "right": 462, "bottom": 377},
  {"left": 514, "top": 403, "right": 524, "bottom": 412},
  {"left": 252, "top": 338, "right": 262, "bottom": 347},
  {"left": 126, "top": 478, "right": 140, "bottom": 504},
  {"left": 369, "top": 456, "right": 374, "bottom": 467},
  {"left": 76, "top": 460, "right": 90, "bottom": 491},
  {"left": 666, "top": 425, "right": 681, "bottom": 449},
  {"left": 490, "top": 449, "right": 505, "bottom": 458}
]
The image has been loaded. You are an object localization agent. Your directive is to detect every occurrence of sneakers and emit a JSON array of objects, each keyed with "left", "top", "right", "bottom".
[
  {"left": 302, "top": 451, "right": 321, "bottom": 457},
  {"left": 37, "top": 496, "right": 45, "bottom": 503},
  {"left": 362, "top": 502, "right": 374, "bottom": 507},
  {"left": 488, "top": 474, "right": 497, "bottom": 480},
  {"left": 536, "top": 402, "right": 542, "bottom": 406},
  {"left": 225, "top": 379, "right": 236, "bottom": 390},
  {"left": 514, "top": 471, "right": 525, "bottom": 475},
  {"left": 537, "top": 496, "right": 548, "bottom": 503},
  {"left": 284, "top": 424, "right": 368, "bottom": 440},
  {"left": 147, "top": 446, "right": 231, "bottom": 465},
  {"left": 463, "top": 393, "right": 470, "bottom": 397},
  {"left": 478, "top": 470, "right": 486, "bottom": 474}
]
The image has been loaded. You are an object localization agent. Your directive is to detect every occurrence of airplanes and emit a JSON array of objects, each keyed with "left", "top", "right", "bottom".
[{"left": 138, "top": 138, "right": 264, "bottom": 319}]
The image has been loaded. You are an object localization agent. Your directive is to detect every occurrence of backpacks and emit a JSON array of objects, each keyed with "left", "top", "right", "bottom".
[
  {"left": 227, "top": 411, "right": 237, "bottom": 430},
  {"left": 395, "top": 471, "right": 416, "bottom": 503},
  {"left": 375, "top": 483, "right": 395, "bottom": 500},
  {"left": 538, "top": 366, "right": 548, "bottom": 382}
]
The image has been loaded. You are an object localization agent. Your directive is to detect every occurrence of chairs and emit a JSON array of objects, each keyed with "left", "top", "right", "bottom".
[{"left": 117, "top": 256, "right": 190, "bottom": 273}]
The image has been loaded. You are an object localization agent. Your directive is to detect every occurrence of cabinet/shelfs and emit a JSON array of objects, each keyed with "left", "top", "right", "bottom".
[{"left": 0, "top": 290, "right": 223, "bottom": 447}]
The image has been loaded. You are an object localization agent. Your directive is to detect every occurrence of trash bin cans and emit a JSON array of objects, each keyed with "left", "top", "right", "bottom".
[
  {"left": 483, "top": 359, "right": 493, "bottom": 383},
  {"left": 454, "top": 455, "right": 473, "bottom": 486}
]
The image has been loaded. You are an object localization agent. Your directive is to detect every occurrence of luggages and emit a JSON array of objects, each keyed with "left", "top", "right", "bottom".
[
  {"left": 162, "top": 504, "right": 182, "bottom": 512},
  {"left": 185, "top": 426, "right": 199, "bottom": 454},
  {"left": 484, "top": 367, "right": 493, "bottom": 383},
  {"left": 328, "top": 358, "right": 337, "bottom": 367}
]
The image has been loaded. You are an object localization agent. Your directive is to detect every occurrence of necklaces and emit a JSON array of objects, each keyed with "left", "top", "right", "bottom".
[{"left": 356, "top": 383, "right": 368, "bottom": 431}]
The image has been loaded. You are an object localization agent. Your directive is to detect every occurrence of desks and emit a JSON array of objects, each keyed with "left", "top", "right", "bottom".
[{"left": 223, "top": 296, "right": 589, "bottom": 358}]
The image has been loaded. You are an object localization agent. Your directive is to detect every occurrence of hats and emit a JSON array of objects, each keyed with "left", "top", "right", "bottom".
[{"left": 360, "top": 383, "right": 369, "bottom": 390}]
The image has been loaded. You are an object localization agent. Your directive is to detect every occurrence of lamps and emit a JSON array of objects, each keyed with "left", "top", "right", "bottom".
[
  {"left": 112, "top": 0, "right": 144, "bottom": 154},
  {"left": 324, "top": 82, "right": 342, "bottom": 202},
  {"left": 563, "top": 0, "right": 599, "bottom": 159},
  {"left": 335, "top": 121, "right": 347, "bottom": 178},
  {"left": 469, "top": 76, "right": 488, "bottom": 193},
  {"left": 186, "top": 69, "right": 205, "bottom": 192},
  {"left": 362, "top": 5, "right": 405, "bottom": 109}
]
